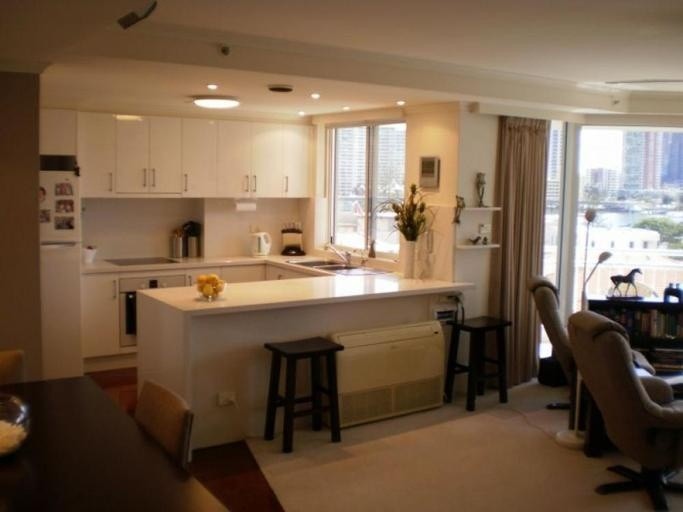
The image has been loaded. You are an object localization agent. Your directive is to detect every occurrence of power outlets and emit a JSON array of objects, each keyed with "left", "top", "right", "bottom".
[{"left": 218, "top": 390, "right": 239, "bottom": 407}]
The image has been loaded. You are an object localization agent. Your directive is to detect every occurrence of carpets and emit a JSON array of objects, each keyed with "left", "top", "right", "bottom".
[{"left": 84, "top": 365, "right": 283, "bottom": 511}]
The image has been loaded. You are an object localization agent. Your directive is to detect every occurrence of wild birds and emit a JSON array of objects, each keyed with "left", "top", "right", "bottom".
[{"left": 469, "top": 236, "right": 481, "bottom": 244}]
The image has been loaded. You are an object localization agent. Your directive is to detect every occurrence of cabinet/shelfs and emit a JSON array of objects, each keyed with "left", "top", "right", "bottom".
[
  {"left": 81, "top": 262, "right": 332, "bottom": 357},
  {"left": 79, "top": 114, "right": 316, "bottom": 200}
]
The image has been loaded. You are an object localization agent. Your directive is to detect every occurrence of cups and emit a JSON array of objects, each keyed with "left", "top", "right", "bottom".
[
  {"left": 171, "top": 235, "right": 198, "bottom": 259},
  {"left": 83, "top": 247, "right": 96, "bottom": 264}
]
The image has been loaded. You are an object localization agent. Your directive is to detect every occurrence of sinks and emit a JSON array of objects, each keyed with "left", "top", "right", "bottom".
[
  {"left": 316, "top": 266, "right": 356, "bottom": 270},
  {"left": 287, "top": 260, "right": 341, "bottom": 267}
]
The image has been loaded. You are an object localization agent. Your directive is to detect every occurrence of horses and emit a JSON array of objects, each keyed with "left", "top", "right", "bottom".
[{"left": 611, "top": 268, "right": 642, "bottom": 297}]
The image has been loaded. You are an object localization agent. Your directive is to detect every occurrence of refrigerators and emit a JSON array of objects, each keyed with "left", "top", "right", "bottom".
[{"left": 39, "top": 168, "right": 83, "bottom": 383}]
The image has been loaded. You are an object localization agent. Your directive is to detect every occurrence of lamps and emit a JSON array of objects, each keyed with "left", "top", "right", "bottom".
[
  {"left": 556, "top": 205, "right": 612, "bottom": 449},
  {"left": 193, "top": 94, "right": 241, "bottom": 112}
]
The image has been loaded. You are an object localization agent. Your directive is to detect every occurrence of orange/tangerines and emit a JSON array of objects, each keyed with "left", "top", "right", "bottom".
[{"left": 197, "top": 274, "right": 224, "bottom": 298}]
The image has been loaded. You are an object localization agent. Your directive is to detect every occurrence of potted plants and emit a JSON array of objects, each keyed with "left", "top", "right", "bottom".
[{"left": 372, "top": 181, "right": 429, "bottom": 279}]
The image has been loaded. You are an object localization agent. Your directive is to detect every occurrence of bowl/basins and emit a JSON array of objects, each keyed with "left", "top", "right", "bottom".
[{"left": 193, "top": 282, "right": 227, "bottom": 301}]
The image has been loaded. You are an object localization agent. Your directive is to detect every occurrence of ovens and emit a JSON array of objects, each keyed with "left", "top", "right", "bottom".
[{"left": 119, "top": 274, "right": 191, "bottom": 347}]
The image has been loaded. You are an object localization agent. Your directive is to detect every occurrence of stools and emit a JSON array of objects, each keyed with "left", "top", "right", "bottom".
[
  {"left": 444, "top": 314, "right": 515, "bottom": 413},
  {"left": 264, "top": 336, "right": 344, "bottom": 455}
]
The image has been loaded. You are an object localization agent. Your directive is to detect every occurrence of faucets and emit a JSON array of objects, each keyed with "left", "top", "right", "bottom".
[{"left": 324, "top": 243, "right": 351, "bottom": 264}]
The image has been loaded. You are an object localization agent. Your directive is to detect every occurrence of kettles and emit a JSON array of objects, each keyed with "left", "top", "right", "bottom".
[{"left": 248, "top": 230, "right": 274, "bottom": 258}]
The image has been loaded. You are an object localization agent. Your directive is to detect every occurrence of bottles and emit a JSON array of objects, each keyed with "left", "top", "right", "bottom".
[{"left": 368, "top": 240, "right": 376, "bottom": 259}]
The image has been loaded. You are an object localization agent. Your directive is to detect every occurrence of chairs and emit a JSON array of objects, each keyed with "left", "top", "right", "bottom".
[
  {"left": 526, "top": 272, "right": 682, "bottom": 511},
  {"left": 130, "top": 380, "right": 205, "bottom": 465}
]
